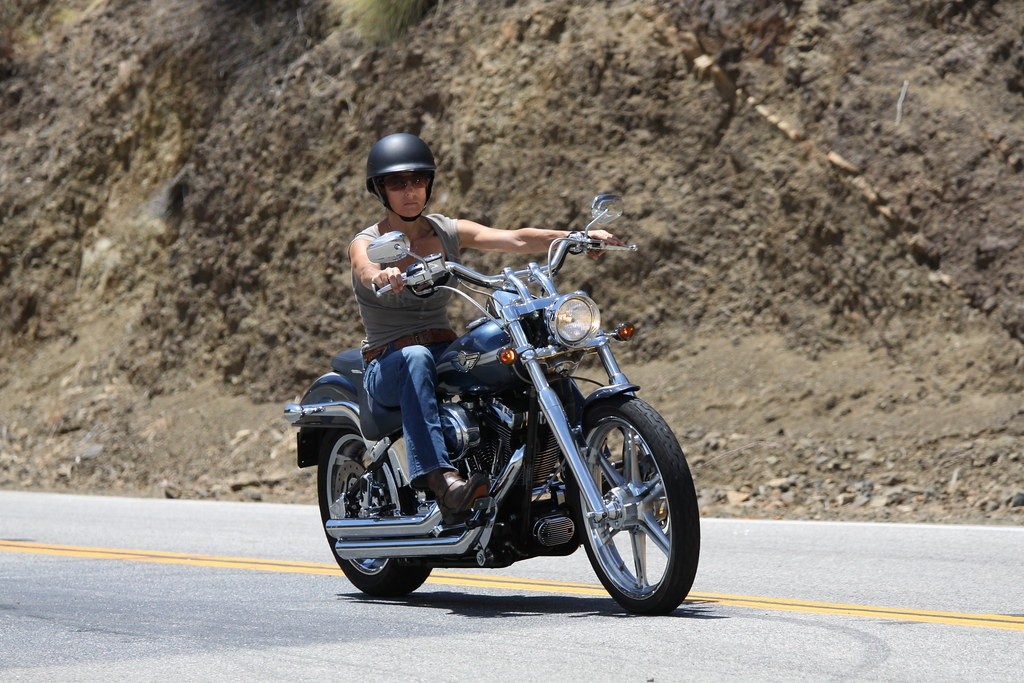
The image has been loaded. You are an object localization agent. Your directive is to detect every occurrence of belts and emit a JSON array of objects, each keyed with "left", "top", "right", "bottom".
[{"left": 366, "top": 329, "right": 458, "bottom": 363}]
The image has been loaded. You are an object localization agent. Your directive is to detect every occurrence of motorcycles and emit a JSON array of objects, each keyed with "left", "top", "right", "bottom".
[{"left": 281, "top": 194, "right": 701, "bottom": 617}]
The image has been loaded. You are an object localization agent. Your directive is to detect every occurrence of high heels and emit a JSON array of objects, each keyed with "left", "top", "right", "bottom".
[{"left": 430, "top": 468, "right": 491, "bottom": 527}]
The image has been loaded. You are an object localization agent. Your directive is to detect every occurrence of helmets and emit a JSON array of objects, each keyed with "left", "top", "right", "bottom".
[{"left": 367, "top": 131, "right": 436, "bottom": 194}]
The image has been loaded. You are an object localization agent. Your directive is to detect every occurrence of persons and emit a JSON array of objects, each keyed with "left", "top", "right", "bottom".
[{"left": 348, "top": 133, "right": 623, "bottom": 525}]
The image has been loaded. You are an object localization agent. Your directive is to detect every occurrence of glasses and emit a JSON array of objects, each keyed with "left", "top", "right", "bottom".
[{"left": 378, "top": 171, "right": 433, "bottom": 190}]
